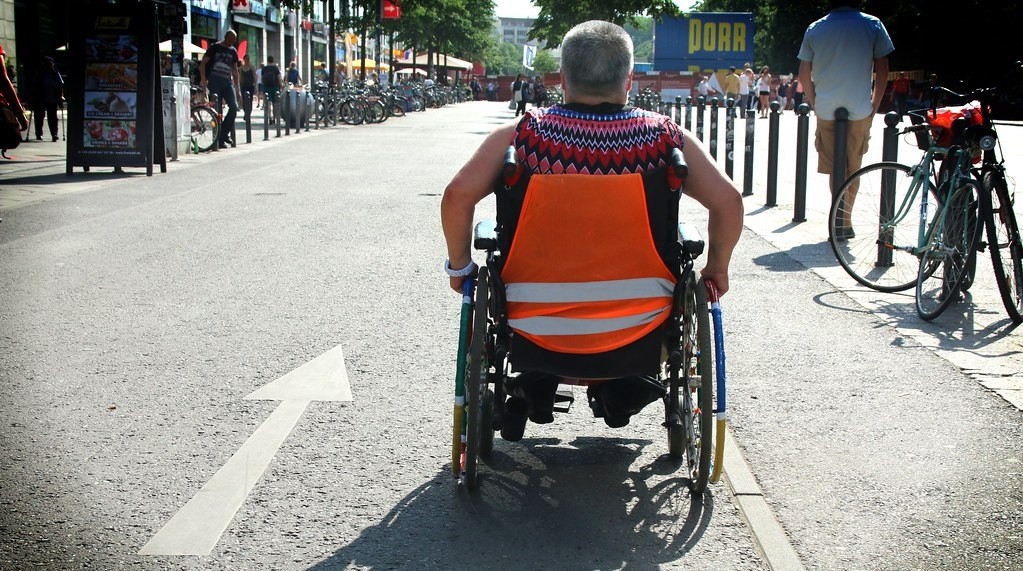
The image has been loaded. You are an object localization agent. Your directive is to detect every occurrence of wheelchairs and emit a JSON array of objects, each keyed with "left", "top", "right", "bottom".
[{"left": 452, "top": 220, "right": 726, "bottom": 498}]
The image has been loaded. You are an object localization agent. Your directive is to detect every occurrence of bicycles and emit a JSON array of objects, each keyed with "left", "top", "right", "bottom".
[
  {"left": 310, "top": 77, "right": 471, "bottom": 124},
  {"left": 189, "top": 86, "right": 222, "bottom": 151},
  {"left": 828, "top": 73, "right": 1023, "bottom": 325}
]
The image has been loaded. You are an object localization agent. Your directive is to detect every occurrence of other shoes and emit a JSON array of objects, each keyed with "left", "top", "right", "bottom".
[
  {"left": 592, "top": 381, "right": 629, "bottom": 428},
  {"left": 520, "top": 374, "right": 556, "bottom": 424},
  {"left": 37, "top": 135, "right": 42, "bottom": 140},
  {"left": 53, "top": 136, "right": 58, "bottom": 142},
  {"left": 844, "top": 227, "right": 855, "bottom": 238},
  {"left": 219, "top": 138, "right": 234, "bottom": 148}
]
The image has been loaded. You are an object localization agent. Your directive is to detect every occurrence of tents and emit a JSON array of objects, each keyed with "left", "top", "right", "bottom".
[{"left": 396, "top": 52, "right": 472, "bottom": 81}]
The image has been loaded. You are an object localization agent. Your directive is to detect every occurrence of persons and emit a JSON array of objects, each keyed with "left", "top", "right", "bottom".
[
  {"left": 798, "top": 0, "right": 895, "bottom": 244},
  {"left": 394, "top": 72, "right": 545, "bottom": 117},
  {"left": 441, "top": 20, "right": 745, "bottom": 428},
  {"left": 200, "top": 31, "right": 242, "bottom": 149},
  {"left": 159, "top": 54, "right": 379, "bottom": 125},
  {"left": 697, "top": 63, "right": 940, "bottom": 123},
  {"left": 0, "top": 43, "right": 64, "bottom": 159}
]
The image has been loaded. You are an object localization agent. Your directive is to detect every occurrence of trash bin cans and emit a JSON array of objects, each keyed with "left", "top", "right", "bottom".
[{"left": 161, "top": 76, "right": 191, "bottom": 156}]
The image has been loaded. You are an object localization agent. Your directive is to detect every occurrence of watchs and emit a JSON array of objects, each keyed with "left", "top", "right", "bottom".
[{"left": 444, "top": 257, "right": 474, "bottom": 277}]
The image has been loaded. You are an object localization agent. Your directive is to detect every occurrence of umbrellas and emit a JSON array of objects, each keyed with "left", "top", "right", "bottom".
[
  {"left": 313, "top": 60, "right": 326, "bottom": 70},
  {"left": 158, "top": 39, "right": 206, "bottom": 54},
  {"left": 336, "top": 57, "right": 390, "bottom": 70}
]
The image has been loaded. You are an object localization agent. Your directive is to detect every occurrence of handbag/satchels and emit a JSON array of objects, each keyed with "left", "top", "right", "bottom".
[
  {"left": 927, "top": 99, "right": 992, "bottom": 165},
  {"left": 509, "top": 98, "right": 516, "bottom": 109},
  {"left": 514, "top": 90, "right": 522, "bottom": 102},
  {"left": 193, "top": 42, "right": 216, "bottom": 85},
  {"left": 0, "top": 103, "right": 22, "bottom": 148}
]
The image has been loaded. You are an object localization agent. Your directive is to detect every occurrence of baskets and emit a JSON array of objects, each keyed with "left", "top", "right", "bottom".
[{"left": 907, "top": 107, "right": 934, "bottom": 152}]
